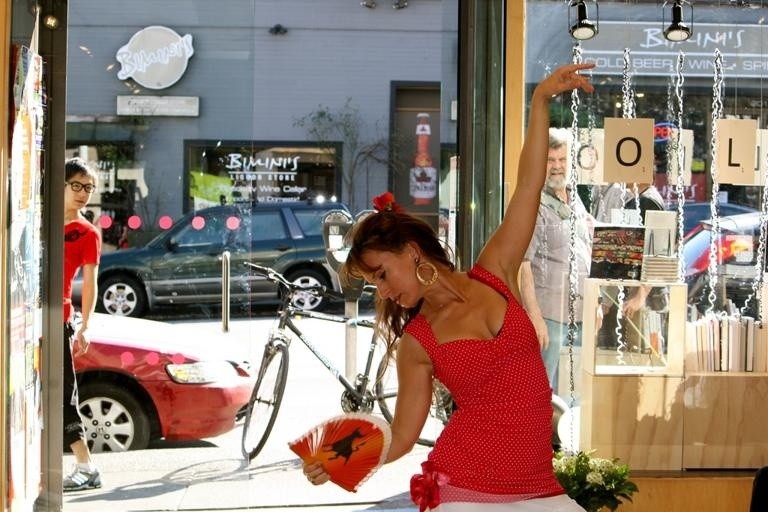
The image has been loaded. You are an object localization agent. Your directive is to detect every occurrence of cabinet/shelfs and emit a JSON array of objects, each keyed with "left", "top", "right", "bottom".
[{"left": 577, "top": 276, "right": 688, "bottom": 475}]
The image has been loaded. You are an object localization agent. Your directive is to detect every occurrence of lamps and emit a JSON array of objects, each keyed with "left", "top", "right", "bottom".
[
  {"left": 566, "top": 1, "right": 599, "bottom": 42},
  {"left": 660, "top": 0, "right": 694, "bottom": 42}
]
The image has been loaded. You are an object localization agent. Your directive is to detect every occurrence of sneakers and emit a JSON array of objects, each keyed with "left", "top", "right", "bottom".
[{"left": 63, "top": 469, "right": 102, "bottom": 492}]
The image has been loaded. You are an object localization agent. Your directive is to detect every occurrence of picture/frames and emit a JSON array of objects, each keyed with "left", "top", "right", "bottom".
[{"left": 641, "top": 209, "right": 678, "bottom": 256}]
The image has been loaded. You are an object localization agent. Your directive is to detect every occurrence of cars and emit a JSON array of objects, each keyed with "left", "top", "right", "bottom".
[
  {"left": 667, "top": 199, "right": 757, "bottom": 234},
  {"left": 658, "top": 211, "right": 768, "bottom": 324},
  {"left": 66, "top": 307, "right": 263, "bottom": 456}
]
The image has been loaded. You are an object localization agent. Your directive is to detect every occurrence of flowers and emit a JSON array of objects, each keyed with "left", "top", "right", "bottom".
[{"left": 549, "top": 447, "right": 639, "bottom": 511}]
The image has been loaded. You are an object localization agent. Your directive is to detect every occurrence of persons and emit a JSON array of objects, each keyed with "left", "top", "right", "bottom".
[
  {"left": 599, "top": 164, "right": 667, "bottom": 352},
  {"left": 518, "top": 128, "right": 598, "bottom": 452},
  {"left": 65, "top": 158, "right": 101, "bottom": 492},
  {"left": 588, "top": 183, "right": 627, "bottom": 346},
  {"left": 303, "top": 62, "right": 596, "bottom": 512}
]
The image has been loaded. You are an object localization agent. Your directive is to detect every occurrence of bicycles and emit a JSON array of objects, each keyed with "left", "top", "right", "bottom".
[{"left": 240, "top": 251, "right": 448, "bottom": 459}]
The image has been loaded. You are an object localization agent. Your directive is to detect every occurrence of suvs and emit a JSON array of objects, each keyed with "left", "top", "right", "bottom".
[{"left": 72, "top": 200, "right": 360, "bottom": 316}]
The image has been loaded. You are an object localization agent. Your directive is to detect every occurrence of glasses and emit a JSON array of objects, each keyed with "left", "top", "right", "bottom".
[{"left": 64, "top": 181, "right": 95, "bottom": 193}]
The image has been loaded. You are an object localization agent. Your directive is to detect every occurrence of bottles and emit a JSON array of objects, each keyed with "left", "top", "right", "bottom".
[{"left": 409, "top": 109, "right": 438, "bottom": 206}]
